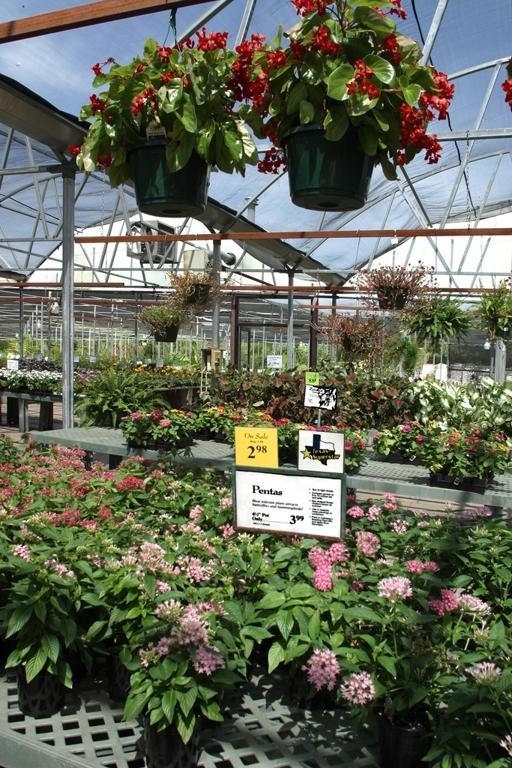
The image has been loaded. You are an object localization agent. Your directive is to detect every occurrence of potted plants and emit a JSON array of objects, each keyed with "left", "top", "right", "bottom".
[
  {"left": 312, "top": 261, "right": 510, "bottom": 351},
  {"left": 141, "top": 305, "right": 185, "bottom": 341},
  {"left": 168, "top": 259, "right": 223, "bottom": 312}
]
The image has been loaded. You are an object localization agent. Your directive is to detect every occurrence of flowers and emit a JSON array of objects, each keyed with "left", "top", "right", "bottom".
[
  {"left": 1, "top": 342, "right": 509, "bottom": 765},
  {"left": 501, "top": 57, "right": 511, "bottom": 115},
  {"left": 235, "top": 0, "right": 453, "bottom": 181},
  {"left": 65, "top": 33, "right": 259, "bottom": 179}
]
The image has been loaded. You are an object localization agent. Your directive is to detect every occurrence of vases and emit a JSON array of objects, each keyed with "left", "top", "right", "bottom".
[
  {"left": 133, "top": 141, "right": 209, "bottom": 217},
  {"left": 282, "top": 130, "right": 371, "bottom": 212}
]
